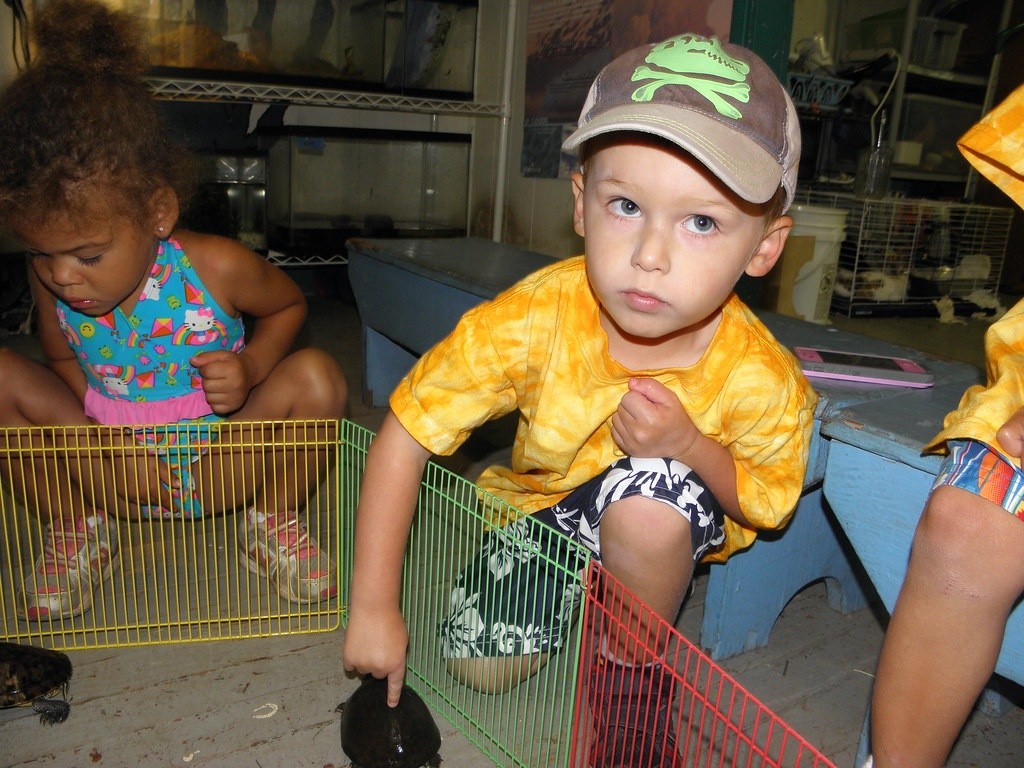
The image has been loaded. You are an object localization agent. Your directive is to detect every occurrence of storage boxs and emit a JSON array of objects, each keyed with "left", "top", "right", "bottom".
[{"left": 842, "top": 7, "right": 971, "bottom": 71}]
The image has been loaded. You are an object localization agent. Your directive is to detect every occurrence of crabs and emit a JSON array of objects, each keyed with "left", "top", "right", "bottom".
[{"left": 31, "top": 699, "right": 71, "bottom": 727}]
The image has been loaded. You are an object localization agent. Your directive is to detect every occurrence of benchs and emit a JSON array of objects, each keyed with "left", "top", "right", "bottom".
[
  {"left": 818, "top": 374, "right": 1024, "bottom": 719},
  {"left": 344, "top": 236, "right": 989, "bottom": 662}
]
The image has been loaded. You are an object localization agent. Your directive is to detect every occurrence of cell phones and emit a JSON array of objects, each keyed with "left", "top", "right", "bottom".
[{"left": 792, "top": 346, "right": 935, "bottom": 388}]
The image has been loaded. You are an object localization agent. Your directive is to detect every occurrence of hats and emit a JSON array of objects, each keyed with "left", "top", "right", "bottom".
[{"left": 560, "top": 33, "right": 801, "bottom": 216}]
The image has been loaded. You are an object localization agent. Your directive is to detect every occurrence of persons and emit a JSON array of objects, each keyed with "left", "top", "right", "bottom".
[
  {"left": 343, "top": 35, "right": 820, "bottom": 768},
  {"left": 860, "top": 85, "right": 1024, "bottom": 768},
  {"left": 0, "top": 0, "right": 349, "bottom": 620}
]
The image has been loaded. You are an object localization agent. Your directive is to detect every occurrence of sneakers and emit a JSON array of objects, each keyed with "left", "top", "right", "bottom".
[
  {"left": 586, "top": 646, "right": 683, "bottom": 768},
  {"left": 15, "top": 508, "right": 124, "bottom": 621},
  {"left": 238, "top": 505, "right": 340, "bottom": 604}
]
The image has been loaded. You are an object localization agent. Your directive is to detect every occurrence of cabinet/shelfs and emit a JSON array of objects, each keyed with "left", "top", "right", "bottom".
[{"left": 812, "top": 61, "right": 1005, "bottom": 184}]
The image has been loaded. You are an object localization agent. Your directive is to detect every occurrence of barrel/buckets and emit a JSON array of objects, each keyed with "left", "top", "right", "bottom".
[{"left": 764, "top": 202, "right": 849, "bottom": 326}]
